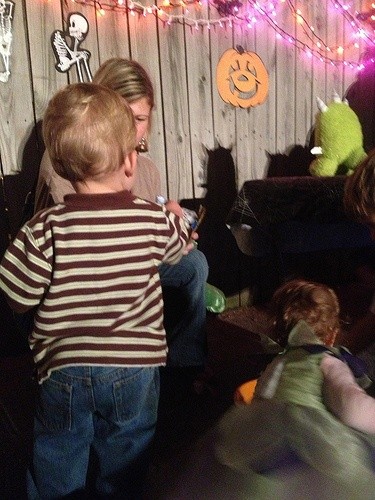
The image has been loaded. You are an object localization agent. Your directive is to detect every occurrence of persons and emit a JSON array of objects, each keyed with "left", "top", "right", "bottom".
[
  {"left": 36, "top": 58, "right": 228, "bottom": 455},
  {"left": 343, "top": 148, "right": 375, "bottom": 226},
  {"left": 0, "top": 83, "right": 193, "bottom": 500},
  {"left": 217, "top": 276, "right": 375, "bottom": 500}
]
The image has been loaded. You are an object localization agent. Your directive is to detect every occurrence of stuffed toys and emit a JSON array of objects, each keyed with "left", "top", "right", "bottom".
[{"left": 308, "top": 87, "right": 368, "bottom": 178}]
